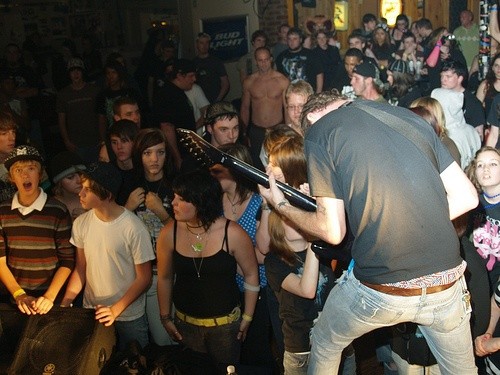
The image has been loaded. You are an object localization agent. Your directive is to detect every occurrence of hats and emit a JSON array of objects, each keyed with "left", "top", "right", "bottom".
[
  {"left": 4, "top": 145, "right": 42, "bottom": 169},
  {"left": 47, "top": 151, "right": 87, "bottom": 183},
  {"left": 206, "top": 102, "right": 238, "bottom": 118},
  {"left": 66, "top": 58, "right": 85, "bottom": 71},
  {"left": 353, "top": 62, "right": 375, "bottom": 78},
  {"left": 376, "top": 23, "right": 389, "bottom": 32}
]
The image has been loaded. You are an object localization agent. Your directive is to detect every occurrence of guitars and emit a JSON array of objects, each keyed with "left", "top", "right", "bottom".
[{"left": 178, "top": 127, "right": 351, "bottom": 272}]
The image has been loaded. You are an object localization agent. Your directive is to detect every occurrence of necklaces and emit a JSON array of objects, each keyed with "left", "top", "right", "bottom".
[
  {"left": 188, "top": 228, "right": 207, "bottom": 252},
  {"left": 191, "top": 246, "right": 207, "bottom": 277},
  {"left": 474, "top": 201, "right": 500, "bottom": 211},
  {"left": 482, "top": 192, "right": 500, "bottom": 198},
  {"left": 186, "top": 222, "right": 205, "bottom": 228},
  {"left": 226, "top": 193, "right": 244, "bottom": 214}
]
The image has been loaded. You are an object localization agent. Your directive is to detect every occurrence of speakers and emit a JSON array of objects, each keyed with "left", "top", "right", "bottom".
[{"left": 0, "top": 302, "right": 117, "bottom": 375}]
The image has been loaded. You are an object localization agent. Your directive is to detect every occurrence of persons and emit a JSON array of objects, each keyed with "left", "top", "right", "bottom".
[{"left": 0, "top": 6, "right": 500, "bottom": 375}]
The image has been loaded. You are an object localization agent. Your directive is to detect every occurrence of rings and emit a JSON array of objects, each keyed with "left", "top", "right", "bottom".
[{"left": 239, "top": 330, "right": 243, "bottom": 333}]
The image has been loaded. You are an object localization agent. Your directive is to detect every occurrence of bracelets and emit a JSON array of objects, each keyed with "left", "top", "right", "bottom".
[
  {"left": 161, "top": 317, "right": 171, "bottom": 322},
  {"left": 13, "top": 288, "right": 27, "bottom": 299},
  {"left": 243, "top": 282, "right": 261, "bottom": 291},
  {"left": 436, "top": 44, "right": 441, "bottom": 48},
  {"left": 161, "top": 215, "right": 171, "bottom": 224},
  {"left": 241, "top": 313, "right": 253, "bottom": 322},
  {"left": 275, "top": 200, "right": 290, "bottom": 211}
]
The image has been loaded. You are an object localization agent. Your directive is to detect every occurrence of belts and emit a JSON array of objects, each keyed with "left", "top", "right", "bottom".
[
  {"left": 361, "top": 275, "right": 464, "bottom": 296},
  {"left": 176, "top": 306, "right": 241, "bottom": 327}
]
